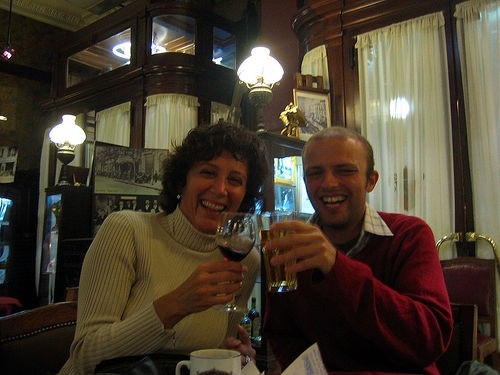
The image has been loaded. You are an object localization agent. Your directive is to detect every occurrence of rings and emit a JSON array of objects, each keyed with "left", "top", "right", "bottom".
[{"left": 245, "top": 355, "right": 251, "bottom": 366}]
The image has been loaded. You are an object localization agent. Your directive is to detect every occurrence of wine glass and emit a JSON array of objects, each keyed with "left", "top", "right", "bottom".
[{"left": 213, "top": 213, "right": 256, "bottom": 311}]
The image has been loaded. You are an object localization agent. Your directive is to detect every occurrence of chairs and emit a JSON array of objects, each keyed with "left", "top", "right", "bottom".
[
  {"left": 441, "top": 256, "right": 500, "bottom": 375},
  {"left": 0, "top": 300, "right": 78, "bottom": 375}
]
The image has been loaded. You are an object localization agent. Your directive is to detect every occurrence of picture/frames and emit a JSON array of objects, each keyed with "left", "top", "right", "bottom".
[{"left": 291, "top": 85, "right": 333, "bottom": 142}]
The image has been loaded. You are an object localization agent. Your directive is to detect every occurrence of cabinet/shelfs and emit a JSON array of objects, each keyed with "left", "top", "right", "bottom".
[{"left": 241, "top": 131, "right": 317, "bottom": 375}]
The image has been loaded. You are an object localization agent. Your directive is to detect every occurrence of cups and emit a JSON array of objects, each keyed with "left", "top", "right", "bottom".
[
  {"left": 258, "top": 211, "right": 298, "bottom": 292},
  {"left": 176, "top": 349, "right": 241, "bottom": 375}
]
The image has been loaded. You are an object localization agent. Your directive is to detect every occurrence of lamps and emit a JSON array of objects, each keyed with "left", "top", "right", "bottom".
[
  {"left": 235, "top": 46, "right": 285, "bottom": 132},
  {"left": 48, "top": 114, "right": 87, "bottom": 187}
]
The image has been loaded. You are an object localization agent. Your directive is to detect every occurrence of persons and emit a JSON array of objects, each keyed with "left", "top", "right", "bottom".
[
  {"left": 95, "top": 168, "right": 161, "bottom": 226},
  {"left": 228, "top": 127, "right": 455, "bottom": 375},
  {"left": 55, "top": 120, "right": 262, "bottom": 375}
]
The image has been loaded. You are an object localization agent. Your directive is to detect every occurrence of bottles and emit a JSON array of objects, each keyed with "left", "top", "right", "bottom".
[
  {"left": 239, "top": 304, "right": 251, "bottom": 340},
  {"left": 248, "top": 297, "right": 260, "bottom": 337}
]
